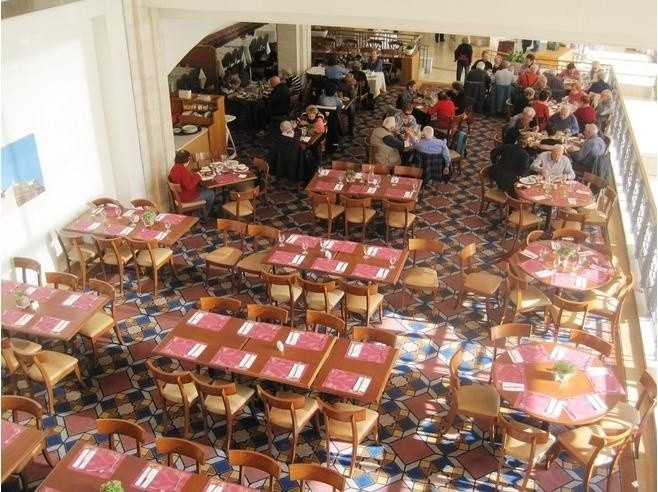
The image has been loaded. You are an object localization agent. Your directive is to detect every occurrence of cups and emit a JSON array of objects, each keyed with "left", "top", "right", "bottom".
[
  {"left": 539, "top": 175, "right": 579, "bottom": 200},
  {"left": 538, "top": 237, "right": 588, "bottom": 271}
]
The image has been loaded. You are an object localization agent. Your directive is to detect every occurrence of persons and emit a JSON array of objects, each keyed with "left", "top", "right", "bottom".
[
  {"left": 491, "top": 83, "right": 614, "bottom": 217},
  {"left": 319, "top": 45, "right": 383, "bottom": 150},
  {"left": 172, "top": 150, "right": 216, "bottom": 219},
  {"left": 454, "top": 37, "right": 610, "bottom": 93},
  {"left": 433, "top": 32, "right": 447, "bottom": 42},
  {"left": 219, "top": 68, "right": 302, "bottom": 125},
  {"left": 370, "top": 78, "right": 464, "bottom": 183},
  {"left": 274, "top": 106, "right": 327, "bottom": 182}
]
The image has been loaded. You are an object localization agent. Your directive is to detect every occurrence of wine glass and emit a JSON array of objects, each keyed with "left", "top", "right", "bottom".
[
  {"left": 86, "top": 200, "right": 172, "bottom": 235},
  {"left": 278, "top": 230, "right": 398, "bottom": 268},
  {"left": 317, "top": 163, "right": 419, "bottom": 196}
]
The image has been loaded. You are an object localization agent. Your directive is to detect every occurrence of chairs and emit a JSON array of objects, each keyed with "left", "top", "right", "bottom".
[
  {"left": 452, "top": 243, "right": 502, "bottom": 325},
  {"left": 546, "top": 295, "right": 588, "bottom": 343},
  {"left": 1, "top": 332, "right": 81, "bottom": 467},
  {"left": 570, "top": 329, "right": 613, "bottom": 361},
  {"left": 401, "top": 237, "right": 444, "bottom": 312},
  {"left": 200, "top": 268, "right": 399, "bottom": 347},
  {"left": 94, "top": 358, "right": 380, "bottom": 491},
  {"left": 598, "top": 369, "right": 657, "bottom": 460},
  {"left": 130, "top": 199, "right": 159, "bottom": 212},
  {"left": 166, "top": 35, "right": 615, "bottom": 251},
  {"left": 504, "top": 262, "right": 552, "bottom": 331},
  {"left": 202, "top": 217, "right": 280, "bottom": 294},
  {"left": 54, "top": 230, "right": 176, "bottom": 296},
  {"left": 488, "top": 323, "right": 532, "bottom": 384},
  {"left": 551, "top": 422, "right": 633, "bottom": 491},
  {"left": 13, "top": 257, "right": 124, "bottom": 354},
  {"left": 498, "top": 410, "right": 557, "bottom": 490},
  {"left": 92, "top": 198, "right": 122, "bottom": 208},
  {"left": 438, "top": 348, "right": 501, "bottom": 442},
  {"left": 588, "top": 272, "right": 635, "bottom": 345}
]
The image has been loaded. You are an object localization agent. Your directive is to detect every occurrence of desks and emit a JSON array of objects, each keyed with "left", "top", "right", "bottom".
[{"left": 491, "top": 341, "right": 623, "bottom": 432}]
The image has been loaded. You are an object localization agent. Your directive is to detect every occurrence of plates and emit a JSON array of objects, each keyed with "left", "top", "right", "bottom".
[
  {"left": 520, "top": 174, "right": 541, "bottom": 186},
  {"left": 196, "top": 159, "right": 250, "bottom": 181},
  {"left": 519, "top": 131, "right": 583, "bottom": 152},
  {"left": 173, "top": 124, "right": 197, "bottom": 133}
]
